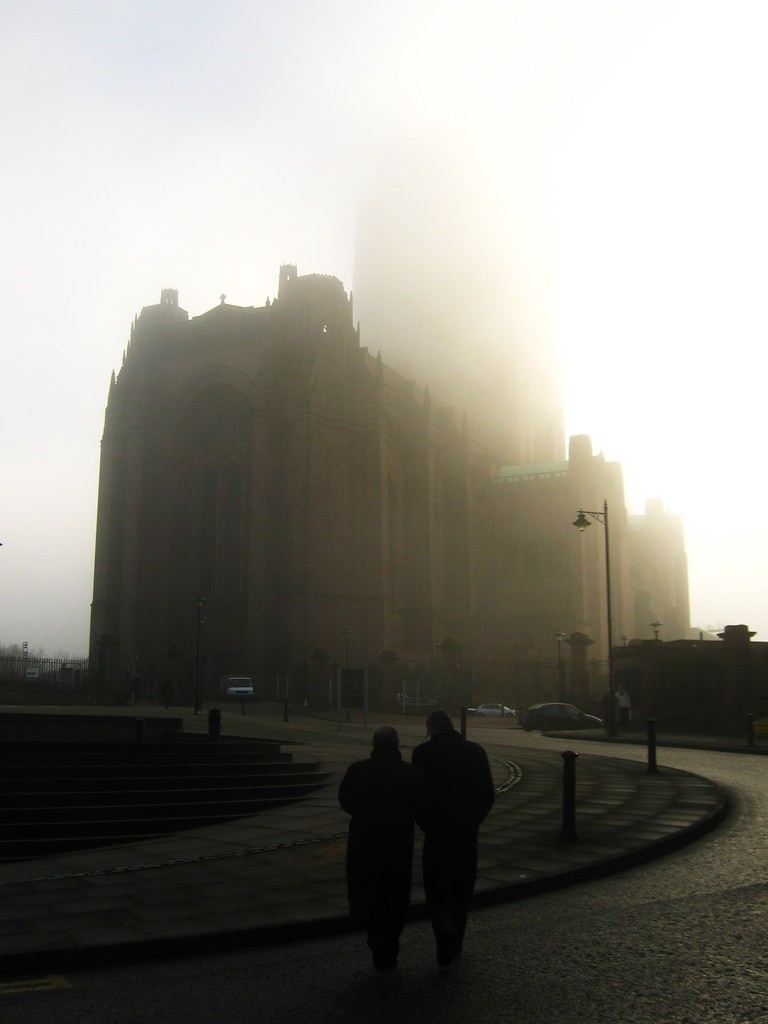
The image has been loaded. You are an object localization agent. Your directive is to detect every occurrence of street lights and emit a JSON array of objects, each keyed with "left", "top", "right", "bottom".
[
  {"left": 648, "top": 620, "right": 663, "bottom": 656},
  {"left": 193, "top": 594, "right": 207, "bottom": 714},
  {"left": 342, "top": 629, "right": 354, "bottom": 720},
  {"left": 574, "top": 499, "right": 617, "bottom": 736},
  {"left": 555, "top": 632, "right": 565, "bottom": 699}
]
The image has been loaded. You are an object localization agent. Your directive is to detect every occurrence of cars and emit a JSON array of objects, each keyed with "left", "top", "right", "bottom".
[
  {"left": 405, "top": 697, "right": 437, "bottom": 705},
  {"left": 519, "top": 702, "right": 604, "bottom": 731},
  {"left": 475, "top": 703, "right": 516, "bottom": 718}
]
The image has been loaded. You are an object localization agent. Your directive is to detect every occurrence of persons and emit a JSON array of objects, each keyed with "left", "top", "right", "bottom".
[
  {"left": 615, "top": 684, "right": 633, "bottom": 732},
  {"left": 131, "top": 675, "right": 142, "bottom": 702},
  {"left": 337, "top": 708, "right": 493, "bottom": 981},
  {"left": 160, "top": 683, "right": 172, "bottom": 709}
]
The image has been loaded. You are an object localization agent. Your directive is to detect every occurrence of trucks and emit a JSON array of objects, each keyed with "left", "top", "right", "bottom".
[{"left": 218, "top": 674, "right": 254, "bottom": 698}]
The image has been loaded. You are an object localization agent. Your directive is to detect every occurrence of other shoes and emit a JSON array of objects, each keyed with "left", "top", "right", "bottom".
[
  {"left": 437, "top": 928, "right": 464, "bottom": 967},
  {"left": 373, "top": 939, "right": 400, "bottom": 972}
]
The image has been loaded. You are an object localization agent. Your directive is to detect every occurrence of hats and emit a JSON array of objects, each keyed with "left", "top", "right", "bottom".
[{"left": 373, "top": 726, "right": 398, "bottom": 748}]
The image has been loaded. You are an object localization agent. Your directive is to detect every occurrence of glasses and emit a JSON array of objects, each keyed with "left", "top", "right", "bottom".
[{"left": 426, "top": 731, "right": 431, "bottom": 741}]
